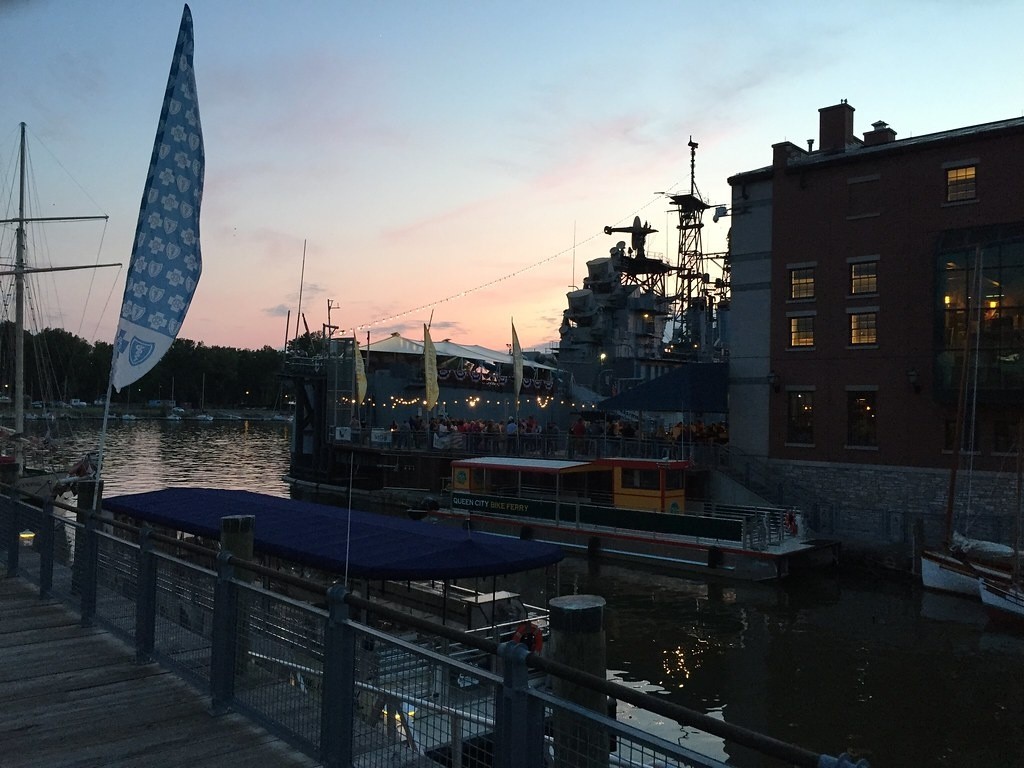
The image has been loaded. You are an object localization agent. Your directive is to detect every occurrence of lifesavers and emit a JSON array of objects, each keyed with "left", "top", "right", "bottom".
[
  {"left": 784, "top": 508, "right": 799, "bottom": 538},
  {"left": 510, "top": 620, "right": 547, "bottom": 659}
]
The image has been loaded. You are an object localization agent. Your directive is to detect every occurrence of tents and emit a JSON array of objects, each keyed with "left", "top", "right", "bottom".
[{"left": 596, "top": 362, "right": 729, "bottom": 459}]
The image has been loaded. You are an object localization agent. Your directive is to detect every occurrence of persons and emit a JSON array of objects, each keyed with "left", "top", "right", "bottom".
[
  {"left": 348, "top": 415, "right": 728, "bottom": 461},
  {"left": 786, "top": 509, "right": 798, "bottom": 536}
]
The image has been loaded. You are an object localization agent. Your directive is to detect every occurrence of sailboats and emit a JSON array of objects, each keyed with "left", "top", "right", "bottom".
[
  {"left": 913, "top": 239, "right": 1024, "bottom": 618},
  {"left": 0, "top": 2, "right": 218, "bottom": 499}
]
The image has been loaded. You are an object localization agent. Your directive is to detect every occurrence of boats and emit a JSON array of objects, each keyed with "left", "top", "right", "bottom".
[
  {"left": 271, "top": 241, "right": 842, "bottom": 583},
  {"left": 571, "top": 134, "right": 732, "bottom": 434},
  {"left": 65, "top": 483, "right": 623, "bottom": 755}
]
